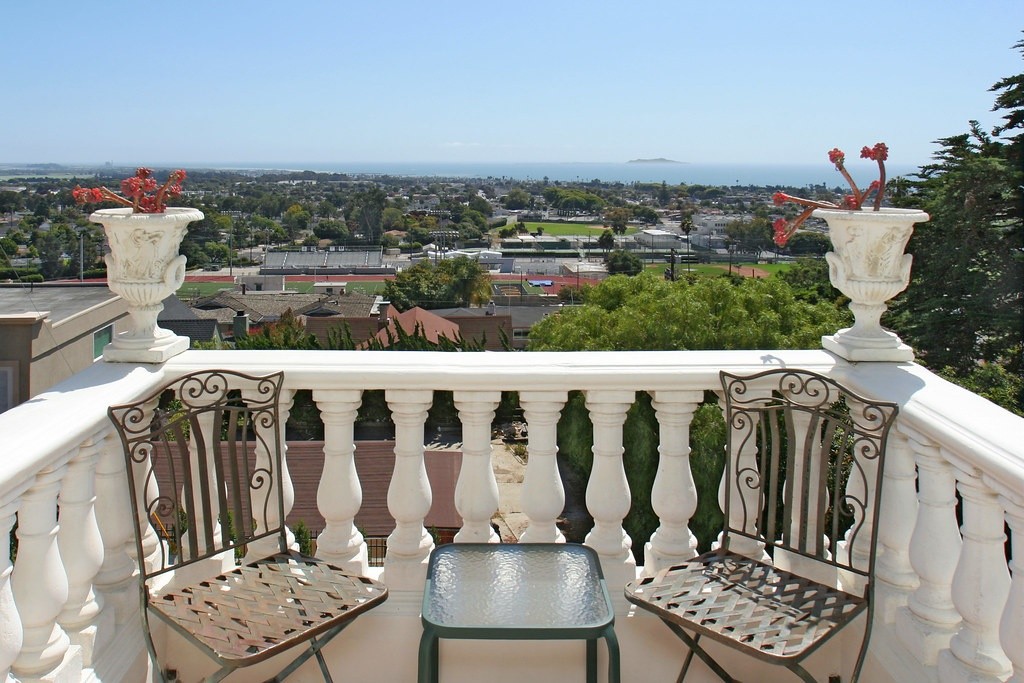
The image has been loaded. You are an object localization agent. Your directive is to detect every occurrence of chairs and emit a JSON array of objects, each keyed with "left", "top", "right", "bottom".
[
  {"left": 106, "top": 369, "right": 391, "bottom": 683},
  {"left": 626, "top": 370, "right": 900, "bottom": 683}
]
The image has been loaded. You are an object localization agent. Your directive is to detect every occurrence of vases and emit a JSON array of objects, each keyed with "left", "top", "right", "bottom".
[
  {"left": 88, "top": 206, "right": 204, "bottom": 348},
  {"left": 813, "top": 206, "right": 931, "bottom": 350}
]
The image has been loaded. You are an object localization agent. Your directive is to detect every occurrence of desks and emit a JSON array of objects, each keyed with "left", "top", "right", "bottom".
[{"left": 417, "top": 542, "right": 620, "bottom": 683}]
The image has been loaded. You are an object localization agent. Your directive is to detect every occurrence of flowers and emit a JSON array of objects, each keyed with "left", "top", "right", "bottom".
[
  {"left": 73, "top": 169, "right": 187, "bottom": 219},
  {"left": 770, "top": 142, "right": 890, "bottom": 249}
]
